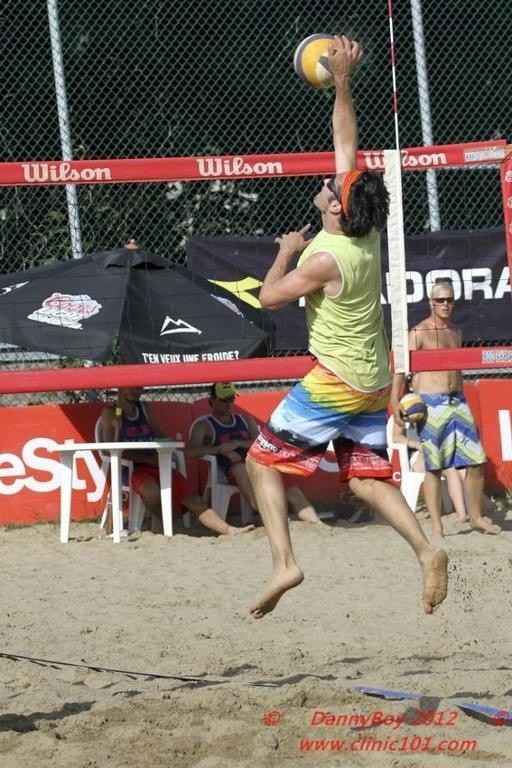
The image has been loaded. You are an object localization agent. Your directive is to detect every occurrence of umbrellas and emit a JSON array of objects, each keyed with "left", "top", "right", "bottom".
[{"left": 0, "top": 240, "right": 270, "bottom": 365}]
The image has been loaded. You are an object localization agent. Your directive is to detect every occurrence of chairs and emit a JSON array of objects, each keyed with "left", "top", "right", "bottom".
[
  {"left": 387, "top": 414, "right": 446, "bottom": 516},
  {"left": 189, "top": 414, "right": 254, "bottom": 531},
  {"left": 94, "top": 409, "right": 191, "bottom": 536}
]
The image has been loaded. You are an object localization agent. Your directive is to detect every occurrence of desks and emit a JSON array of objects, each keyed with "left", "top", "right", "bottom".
[{"left": 51, "top": 441, "right": 185, "bottom": 544}]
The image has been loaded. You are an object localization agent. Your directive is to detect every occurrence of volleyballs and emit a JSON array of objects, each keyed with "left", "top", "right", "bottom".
[
  {"left": 399, "top": 393, "right": 425, "bottom": 422},
  {"left": 293, "top": 33, "right": 335, "bottom": 89}
]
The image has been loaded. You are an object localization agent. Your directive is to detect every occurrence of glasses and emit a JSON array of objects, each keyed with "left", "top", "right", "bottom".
[
  {"left": 432, "top": 298, "right": 453, "bottom": 303},
  {"left": 327, "top": 176, "right": 342, "bottom": 204}
]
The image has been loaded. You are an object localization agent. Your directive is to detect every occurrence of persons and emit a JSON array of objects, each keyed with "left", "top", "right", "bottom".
[
  {"left": 246, "top": 35, "right": 450, "bottom": 624},
  {"left": 100, "top": 387, "right": 256, "bottom": 541},
  {"left": 391, "top": 282, "right": 503, "bottom": 535},
  {"left": 386, "top": 381, "right": 467, "bottom": 524},
  {"left": 186, "top": 385, "right": 327, "bottom": 525}
]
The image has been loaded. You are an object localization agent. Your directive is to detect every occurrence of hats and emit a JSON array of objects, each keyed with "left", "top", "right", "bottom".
[{"left": 211, "top": 382, "right": 240, "bottom": 401}]
